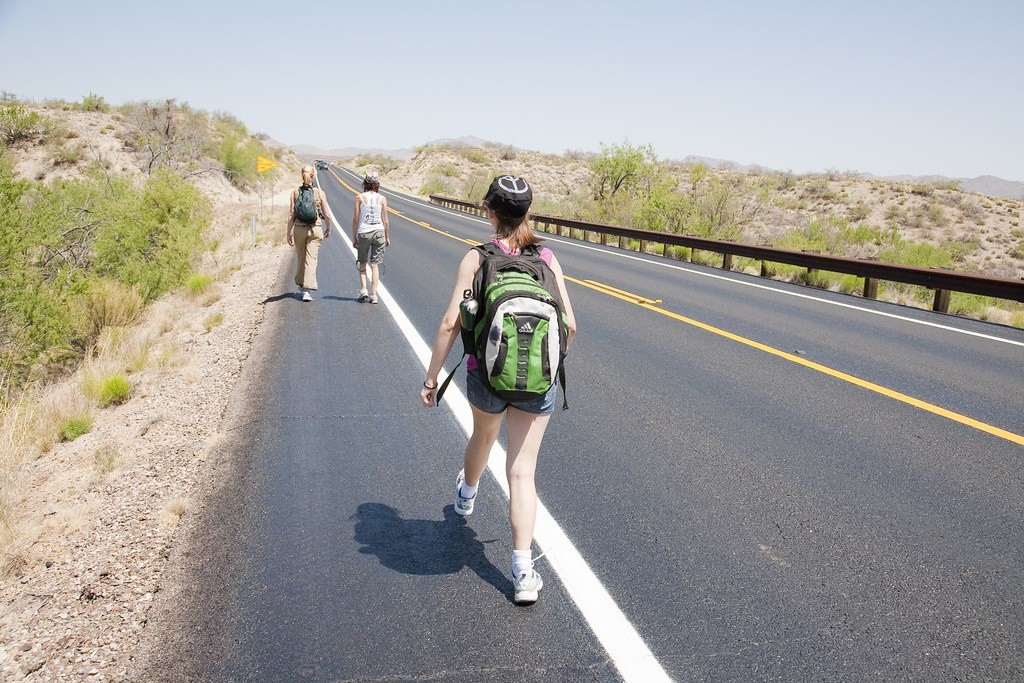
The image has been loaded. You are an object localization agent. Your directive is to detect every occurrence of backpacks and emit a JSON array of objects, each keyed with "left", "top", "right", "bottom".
[
  {"left": 437, "top": 242, "right": 570, "bottom": 411},
  {"left": 294, "top": 185, "right": 318, "bottom": 224}
]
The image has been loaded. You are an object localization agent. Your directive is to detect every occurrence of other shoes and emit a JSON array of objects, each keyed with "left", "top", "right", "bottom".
[
  {"left": 302, "top": 291, "right": 314, "bottom": 300},
  {"left": 358, "top": 289, "right": 368, "bottom": 302},
  {"left": 370, "top": 295, "right": 378, "bottom": 304}
]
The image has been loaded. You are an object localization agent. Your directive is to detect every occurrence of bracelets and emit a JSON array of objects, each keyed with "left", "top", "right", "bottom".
[{"left": 423, "top": 381, "right": 438, "bottom": 389}]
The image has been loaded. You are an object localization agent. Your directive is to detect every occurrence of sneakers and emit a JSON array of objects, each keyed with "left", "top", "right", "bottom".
[
  {"left": 512, "top": 552, "right": 543, "bottom": 602},
  {"left": 455, "top": 468, "right": 480, "bottom": 515}
]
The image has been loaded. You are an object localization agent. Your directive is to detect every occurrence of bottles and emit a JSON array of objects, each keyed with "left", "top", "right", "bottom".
[{"left": 459, "top": 289, "right": 479, "bottom": 331}]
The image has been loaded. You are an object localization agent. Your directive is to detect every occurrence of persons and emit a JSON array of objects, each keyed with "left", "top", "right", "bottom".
[
  {"left": 287, "top": 165, "right": 330, "bottom": 301},
  {"left": 353, "top": 172, "right": 389, "bottom": 303},
  {"left": 420, "top": 175, "right": 575, "bottom": 604}
]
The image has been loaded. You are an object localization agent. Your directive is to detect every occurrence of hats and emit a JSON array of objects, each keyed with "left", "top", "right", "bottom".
[
  {"left": 483, "top": 174, "right": 533, "bottom": 217},
  {"left": 364, "top": 171, "right": 379, "bottom": 183}
]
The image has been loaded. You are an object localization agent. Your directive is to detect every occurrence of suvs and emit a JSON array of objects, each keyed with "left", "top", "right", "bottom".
[{"left": 315, "top": 160, "right": 328, "bottom": 170}]
